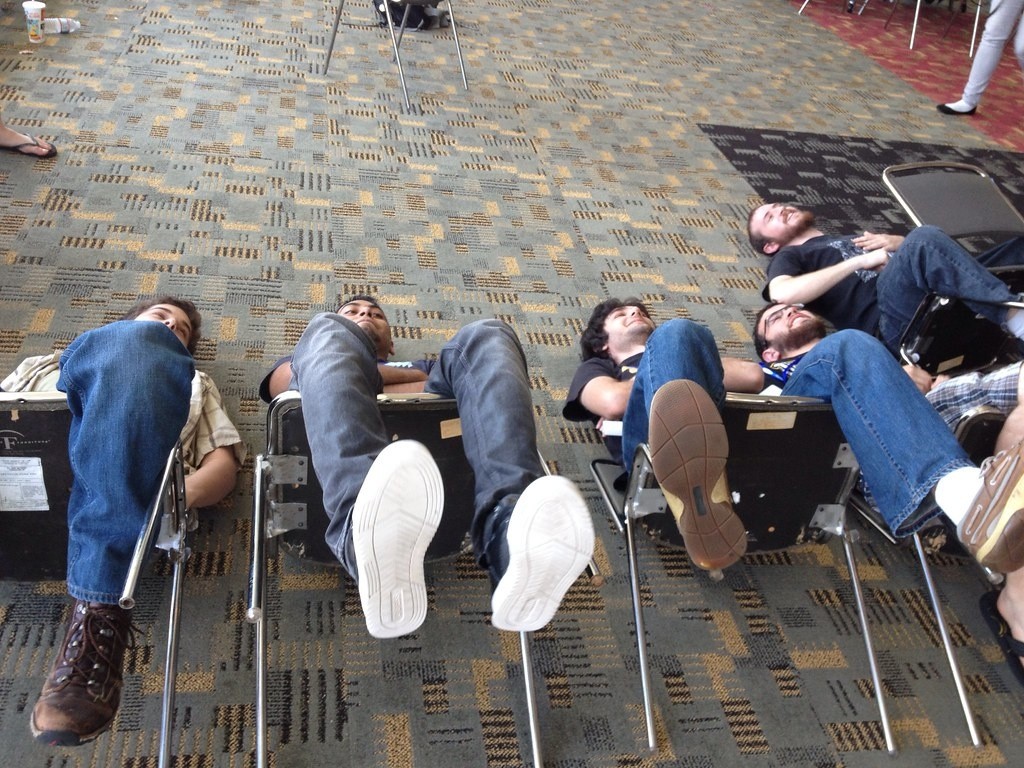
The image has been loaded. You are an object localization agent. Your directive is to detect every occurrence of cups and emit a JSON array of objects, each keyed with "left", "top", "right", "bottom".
[{"left": 22, "top": 1, "right": 46, "bottom": 43}]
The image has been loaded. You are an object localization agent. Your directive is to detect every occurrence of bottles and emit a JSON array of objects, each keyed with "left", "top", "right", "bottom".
[{"left": 44, "top": 18, "right": 81, "bottom": 33}]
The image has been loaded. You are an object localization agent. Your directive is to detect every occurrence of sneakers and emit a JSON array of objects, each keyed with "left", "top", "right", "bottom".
[
  {"left": 954, "top": 434, "right": 1024, "bottom": 573},
  {"left": 29, "top": 597, "right": 144, "bottom": 747}
]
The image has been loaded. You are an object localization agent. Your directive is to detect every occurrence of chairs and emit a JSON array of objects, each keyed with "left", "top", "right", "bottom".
[
  {"left": 0, "top": 159, "right": 1024, "bottom": 768},
  {"left": 322, "top": 0, "right": 469, "bottom": 108}
]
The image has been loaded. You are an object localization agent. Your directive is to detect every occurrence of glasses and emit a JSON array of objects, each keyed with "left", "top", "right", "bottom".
[{"left": 762, "top": 303, "right": 805, "bottom": 349}]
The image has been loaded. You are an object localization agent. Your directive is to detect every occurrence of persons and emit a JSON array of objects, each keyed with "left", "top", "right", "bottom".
[
  {"left": 2, "top": 296, "right": 250, "bottom": 743},
  {"left": 260, "top": 293, "right": 595, "bottom": 639},
  {"left": 563, "top": 297, "right": 1024, "bottom": 575},
  {"left": 0, "top": 111, "right": 58, "bottom": 162},
  {"left": 752, "top": 303, "right": 1024, "bottom": 674},
  {"left": 747, "top": 204, "right": 1024, "bottom": 354},
  {"left": 937, "top": 1, "right": 1024, "bottom": 120}
]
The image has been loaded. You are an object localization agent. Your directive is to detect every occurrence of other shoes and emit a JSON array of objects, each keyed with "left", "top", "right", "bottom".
[
  {"left": 936, "top": 98, "right": 977, "bottom": 115},
  {"left": 648, "top": 378, "right": 748, "bottom": 572},
  {"left": 487, "top": 474, "right": 597, "bottom": 631},
  {"left": 344, "top": 438, "right": 446, "bottom": 639}
]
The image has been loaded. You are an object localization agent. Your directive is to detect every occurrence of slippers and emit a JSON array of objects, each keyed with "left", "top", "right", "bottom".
[{"left": 0, "top": 129, "right": 58, "bottom": 159}]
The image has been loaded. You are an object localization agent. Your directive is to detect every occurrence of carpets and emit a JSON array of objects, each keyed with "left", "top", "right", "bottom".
[{"left": 696, "top": 123, "right": 1023, "bottom": 299}]
